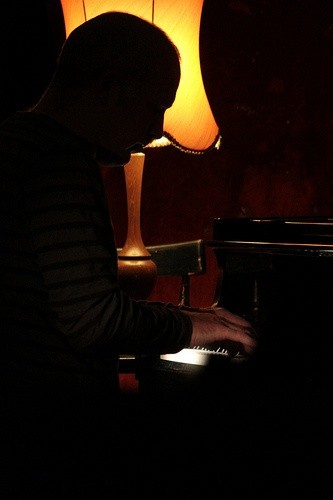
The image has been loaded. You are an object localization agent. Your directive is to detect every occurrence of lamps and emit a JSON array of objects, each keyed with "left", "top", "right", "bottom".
[{"left": 59, "top": 1, "right": 221, "bottom": 280}]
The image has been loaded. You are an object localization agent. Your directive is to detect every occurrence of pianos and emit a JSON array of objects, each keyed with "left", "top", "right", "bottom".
[{"left": 94, "top": 212, "right": 333, "bottom": 466}]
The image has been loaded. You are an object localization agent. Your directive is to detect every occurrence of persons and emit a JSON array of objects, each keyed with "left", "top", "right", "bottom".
[{"left": 1, "top": 12, "right": 266, "bottom": 500}]
